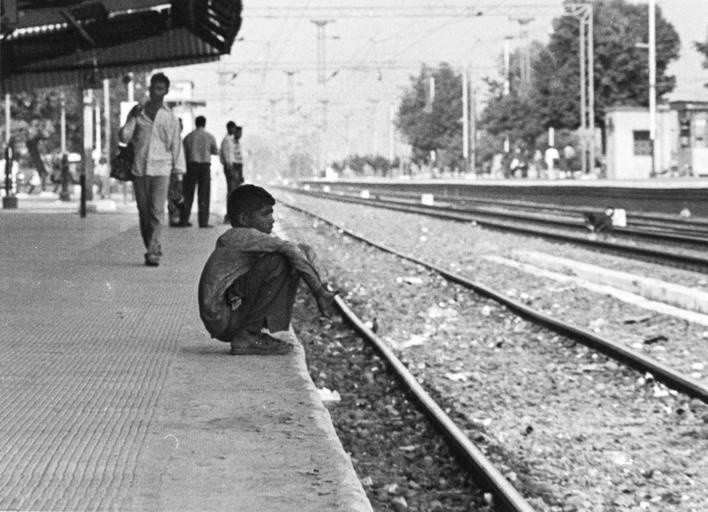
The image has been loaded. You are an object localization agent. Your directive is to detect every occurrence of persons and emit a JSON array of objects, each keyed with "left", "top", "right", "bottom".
[
  {"left": 198, "top": 185, "right": 339, "bottom": 355},
  {"left": 118, "top": 73, "right": 243, "bottom": 266},
  {"left": 93, "top": 157, "right": 108, "bottom": 199}
]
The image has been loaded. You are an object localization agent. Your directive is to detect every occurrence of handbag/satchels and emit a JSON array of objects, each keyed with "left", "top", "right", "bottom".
[{"left": 108, "top": 144, "right": 137, "bottom": 182}]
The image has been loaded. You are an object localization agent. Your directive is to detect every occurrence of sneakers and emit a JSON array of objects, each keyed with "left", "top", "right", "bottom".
[
  {"left": 143, "top": 248, "right": 162, "bottom": 266},
  {"left": 230, "top": 332, "right": 295, "bottom": 355}
]
